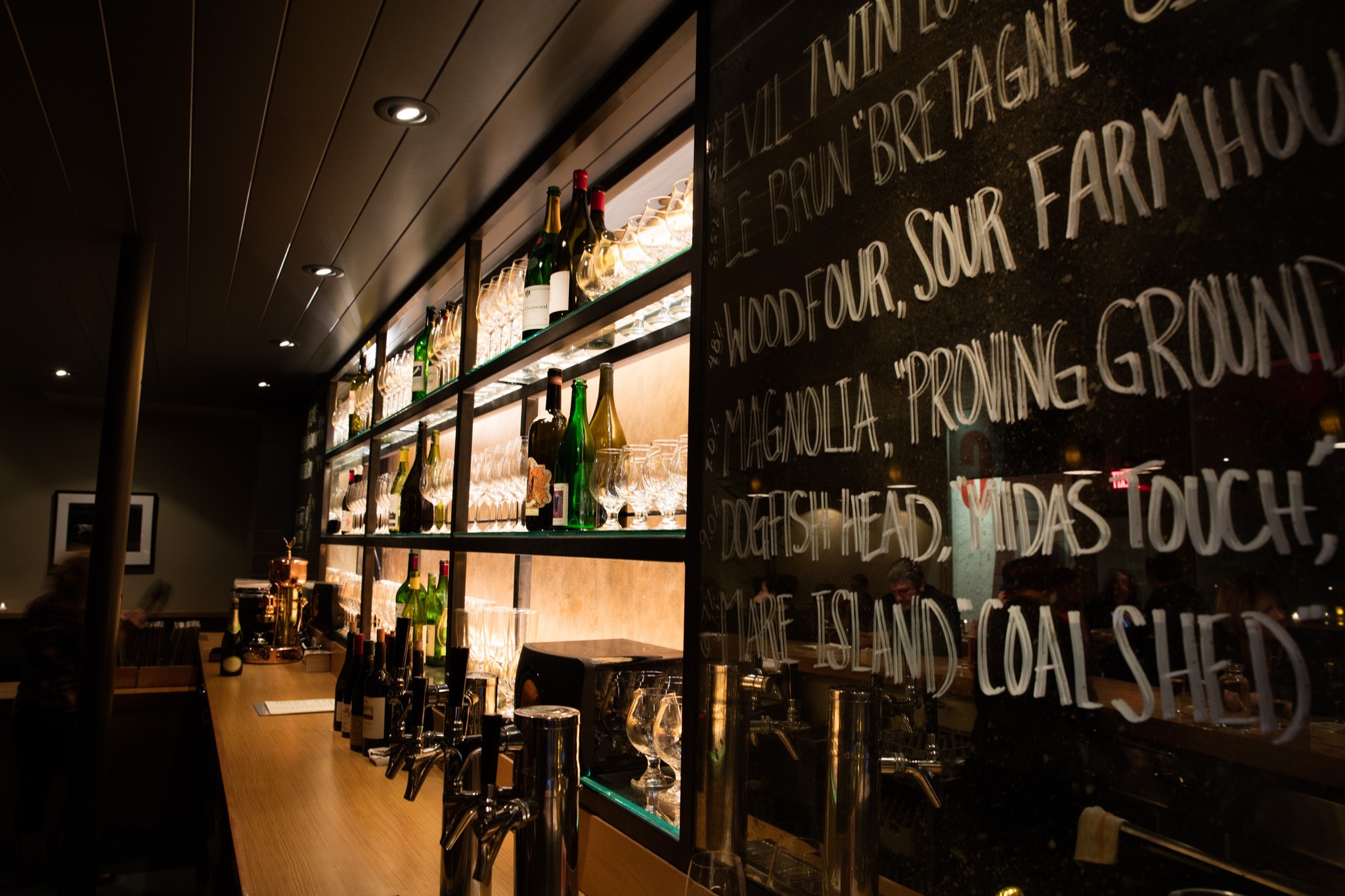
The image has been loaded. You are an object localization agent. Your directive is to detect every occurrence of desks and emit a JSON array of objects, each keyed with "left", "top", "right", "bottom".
[{"left": 196, "top": 631, "right": 709, "bottom": 894}]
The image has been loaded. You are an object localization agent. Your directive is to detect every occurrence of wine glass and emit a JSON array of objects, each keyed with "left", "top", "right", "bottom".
[{"left": 328, "top": 169, "right": 694, "bottom": 824}]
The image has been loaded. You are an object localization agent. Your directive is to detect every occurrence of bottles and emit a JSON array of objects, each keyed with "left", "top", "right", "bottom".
[{"left": 219, "top": 598, "right": 244, "bottom": 677}]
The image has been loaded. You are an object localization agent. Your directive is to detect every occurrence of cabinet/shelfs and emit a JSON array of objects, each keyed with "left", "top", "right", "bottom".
[{"left": 308, "top": 7, "right": 692, "bottom": 872}]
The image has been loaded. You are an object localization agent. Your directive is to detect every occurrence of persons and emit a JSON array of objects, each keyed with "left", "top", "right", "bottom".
[
  {"left": 705, "top": 544, "right": 1345, "bottom": 895},
  {"left": 1, "top": 554, "right": 147, "bottom": 874}
]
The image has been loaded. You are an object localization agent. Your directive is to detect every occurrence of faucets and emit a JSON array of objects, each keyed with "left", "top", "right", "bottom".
[
  {"left": 747, "top": 712, "right": 802, "bottom": 760},
  {"left": 404, "top": 725, "right": 448, "bottom": 802},
  {"left": 740, "top": 662, "right": 790, "bottom": 702},
  {"left": 385, "top": 703, "right": 443, "bottom": 780},
  {"left": 472, "top": 784, "right": 531, "bottom": 886},
  {"left": 882, "top": 675, "right": 922, "bottom": 733},
  {"left": 440, "top": 775, "right": 482, "bottom": 851},
  {"left": 879, "top": 730, "right": 946, "bottom": 809}
]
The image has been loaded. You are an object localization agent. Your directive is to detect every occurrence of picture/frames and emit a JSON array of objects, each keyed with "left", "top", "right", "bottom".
[{"left": 47, "top": 489, "right": 159, "bottom": 575}]
